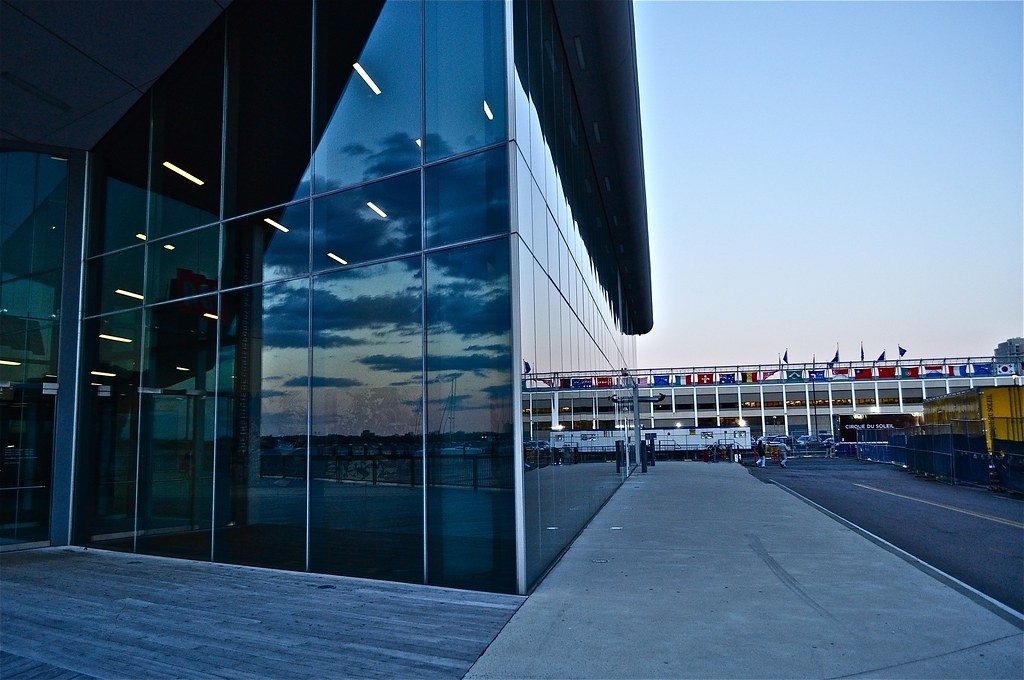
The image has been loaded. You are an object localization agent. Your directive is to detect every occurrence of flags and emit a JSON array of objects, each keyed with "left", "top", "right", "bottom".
[
  {"left": 876, "top": 349, "right": 885, "bottom": 363},
  {"left": 899, "top": 346, "right": 906, "bottom": 357},
  {"left": 638, "top": 361, "right": 1015, "bottom": 386},
  {"left": 783, "top": 348, "right": 788, "bottom": 364},
  {"left": 829, "top": 350, "right": 838, "bottom": 368},
  {"left": 523, "top": 359, "right": 531, "bottom": 373},
  {"left": 860, "top": 341, "right": 864, "bottom": 360}
]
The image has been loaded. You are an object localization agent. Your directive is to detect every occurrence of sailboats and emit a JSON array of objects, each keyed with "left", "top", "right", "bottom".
[{"left": 415, "top": 377, "right": 486, "bottom": 458}]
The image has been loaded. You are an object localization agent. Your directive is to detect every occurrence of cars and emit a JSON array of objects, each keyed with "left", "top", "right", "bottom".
[
  {"left": 524, "top": 441, "right": 549, "bottom": 451},
  {"left": 751, "top": 433, "right": 834, "bottom": 451}
]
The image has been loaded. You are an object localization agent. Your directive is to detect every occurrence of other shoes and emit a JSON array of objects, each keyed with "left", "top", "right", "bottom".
[
  {"left": 780, "top": 464, "right": 786, "bottom": 468},
  {"left": 755, "top": 463, "right": 758, "bottom": 467}
]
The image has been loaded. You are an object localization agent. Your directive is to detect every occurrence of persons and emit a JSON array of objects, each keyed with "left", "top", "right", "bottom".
[
  {"left": 756, "top": 440, "right": 767, "bottom": 468},
  {"left": 778, "top": 439, "right": 791, "bottom": 468},
  {"left": 646, "top": 444, "right": 651, "bottom": 463},
  {"left": 825, "top": 440, "right": 832, "bottom": 458},
  {"left": 751, "top": 434, "right": 755, "bottom": 445},
  {"left": 703, "top": 442, "right": 732, "bottom": 463}
]
